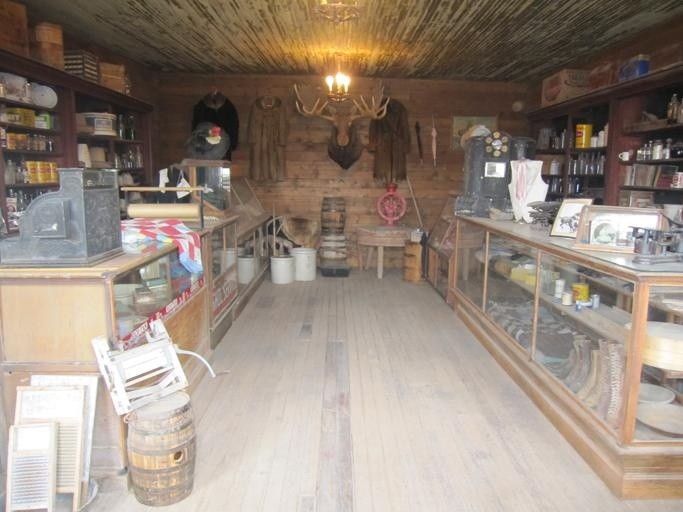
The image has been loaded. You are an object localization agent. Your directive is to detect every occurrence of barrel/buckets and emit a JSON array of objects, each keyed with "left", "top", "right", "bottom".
[
  {"left": 321, "top": 197, "right": 347, "bottom": 235},
  {"left": 219, "top": 247, "right": 246, "bottom": 277},
  {"left": 113, "top": 284, "right": 145, "bottom": 309},
  {"left": 401, "top": 241, "right": 424, "bottom": 284},
  {"left": 238, "top": 254, "right": 258, "bottom": 283},
  {"left": 318, "top": 236, "right": 349, "bottom": 275},
  {"left": 124, "top": 392, "right": 196, "bottom": 507},
  {"left": 290, "top": 247, "right": 317, "bottom": 280},
  {"left": 270, "top": 255, "right": 293, "bottom": 283}
]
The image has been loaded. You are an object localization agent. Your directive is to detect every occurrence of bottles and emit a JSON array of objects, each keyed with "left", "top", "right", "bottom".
[
  {"left": 118, "top": 114, "right": 126, "bottom": 139},
  {"left": 0, "top": 110, "right": 59, "bottom": 213},
  {"left": 127, "top": 116, "right": 136, "bottom": 140},
  {"left": 77, "top": 143, "right": 143, "bottom": 170},
  {"left": 87, "top": 171, "right": 140, "bottom": 212},
  {"left": 534, "top": 95, "right": 682, "bottom": 195},
  {"left": 124, "top": 73, "right": 132, "bottom": 96}
]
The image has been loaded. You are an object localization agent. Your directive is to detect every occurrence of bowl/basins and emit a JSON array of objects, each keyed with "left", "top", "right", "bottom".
[{"left": 113, "top": 284, "right": 143, "bottom": 305}]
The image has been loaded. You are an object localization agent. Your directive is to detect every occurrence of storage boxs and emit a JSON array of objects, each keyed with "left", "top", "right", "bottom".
[{"left": 0, "top": 0, "right": 131, "bottom": 99}]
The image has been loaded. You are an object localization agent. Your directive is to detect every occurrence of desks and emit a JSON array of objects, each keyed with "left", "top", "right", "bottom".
[{"left": 355, "top": 230, "right": 410, "bottom": 280}]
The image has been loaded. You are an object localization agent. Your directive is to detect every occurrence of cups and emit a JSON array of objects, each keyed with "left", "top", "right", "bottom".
[
  {"left": 0, "top": 83, "right": 6, "bottom": 97},
  {"left": 541, "top": 270, "right": 600, "bottom": 313}
]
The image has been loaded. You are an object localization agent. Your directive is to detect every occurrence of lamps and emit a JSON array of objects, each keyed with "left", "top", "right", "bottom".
[{"left": 324, "top": 53, "right": 352, "bottom": 105}]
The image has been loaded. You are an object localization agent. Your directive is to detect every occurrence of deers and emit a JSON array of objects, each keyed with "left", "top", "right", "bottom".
[{"left": 293, "top": 82, "right": 391, "bottom": 148}]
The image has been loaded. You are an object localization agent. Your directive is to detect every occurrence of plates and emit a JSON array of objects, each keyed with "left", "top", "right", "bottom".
[
  {"left": 633, "top": 404, "right": 682, "bottom": 435},
  {"left": 635, "top": 381, "right": 675, "bottom": 404},
  {"left": 21, "top": 83, "right": 38, "bottom": 105},
  {"left": 31, "top": 86, "right": 58, "bottom": 109}
]
